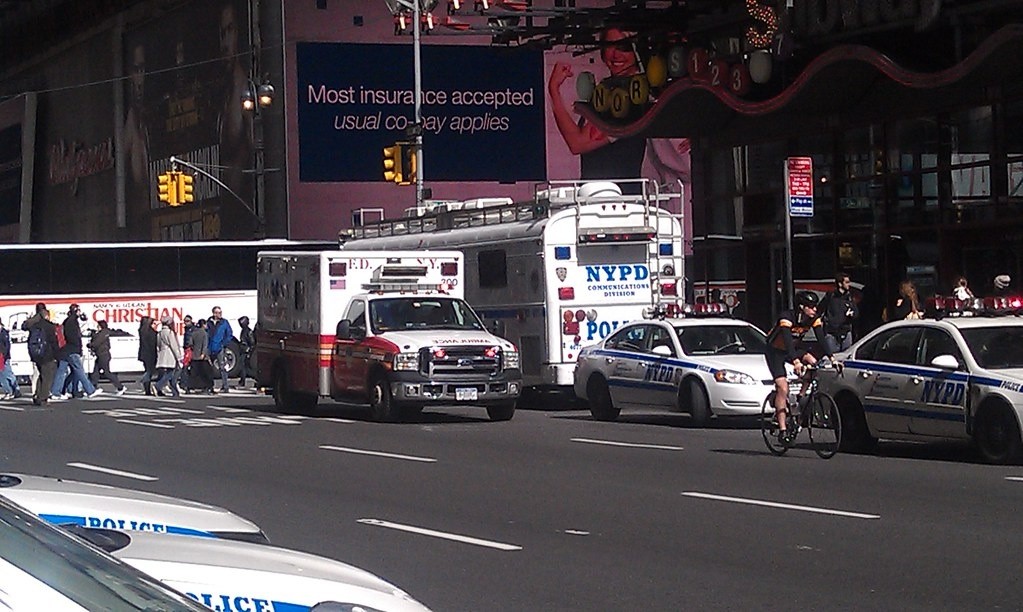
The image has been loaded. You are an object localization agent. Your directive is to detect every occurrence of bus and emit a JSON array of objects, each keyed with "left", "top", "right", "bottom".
[
  {"left": 686, "top": 230, "right": 907, "bottom": 315},
  {"left": 0, "top": 239, "right": 343, "bottom": 377}
]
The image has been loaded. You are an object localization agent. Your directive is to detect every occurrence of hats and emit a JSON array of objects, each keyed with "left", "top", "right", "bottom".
[
  {"left": 162, "top": 316, "right": 172, "bottom": 323},
  {"left": 994, "top": 275, "right": 1010, "bottom": 289}
]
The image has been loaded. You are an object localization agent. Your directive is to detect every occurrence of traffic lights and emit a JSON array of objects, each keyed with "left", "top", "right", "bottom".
[
  {"left": 176, "top": 174, "right": 193, "bottom": 203},
  {"left": 385, "top": 147, "right": 402, "bottom": 183},
  {"left": 158, "top": 174, "right": 176, "bottom": 205}
]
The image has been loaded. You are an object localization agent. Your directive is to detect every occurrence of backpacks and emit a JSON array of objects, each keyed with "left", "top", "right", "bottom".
[{"left": 27, "top": 325, "right": 56, "bottom": 360}]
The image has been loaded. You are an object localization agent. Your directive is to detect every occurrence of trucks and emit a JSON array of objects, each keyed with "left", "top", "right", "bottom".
[{"left": 340, "top": 178, "right": 687, "bottom": 401}]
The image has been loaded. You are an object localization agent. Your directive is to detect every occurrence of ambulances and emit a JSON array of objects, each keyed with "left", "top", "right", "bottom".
[{"left": 255, "top": 250, "right": 523, "bottom": 422}]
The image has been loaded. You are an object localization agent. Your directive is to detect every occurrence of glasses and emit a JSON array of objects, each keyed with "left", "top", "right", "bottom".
[{"left": 805, "top": 304, "right": 819, "bottom": 309}]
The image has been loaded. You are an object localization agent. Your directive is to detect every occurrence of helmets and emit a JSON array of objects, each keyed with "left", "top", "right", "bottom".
[{"left": 794, "top": 291, "right": 819, "bottom": 313}]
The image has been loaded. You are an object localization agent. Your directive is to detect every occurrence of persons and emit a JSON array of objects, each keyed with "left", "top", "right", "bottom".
[
  {"left": 692, "top": 271, "right": 1011, "bottom": 359},
  {"left": 765, "top": 291, "right": 844, "bottom": 445},
  {"left": 0, "top": 302, "right": 257, "bottom": 408},
  {"left": 549, "top": 29, "right": 646, "bottom": 196}
]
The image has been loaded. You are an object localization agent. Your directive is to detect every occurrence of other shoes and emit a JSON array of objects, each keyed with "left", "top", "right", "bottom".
[
  {"left": 235, "top": 384, "right": 243, "bottom": 387},
  {"left": 64, "top": 392, "right": 72, "bottom": 398},
  {"left": 152, "top": 385, "right": 158, "bottom": 397},
  {"left": 88, "top": 387, "right": 103, "bottom": 398},
  {"left": 778, "top": 429, "right": 790, "bottom": 443},
  {"left": 48, "top": 391, "right": 68, "bottom": 400},
  {"left": 0, "top": 393, "right": 14, "bottom": 401},
  {"left": 116, "top": 386, "right": 128, "bottom": 396}
]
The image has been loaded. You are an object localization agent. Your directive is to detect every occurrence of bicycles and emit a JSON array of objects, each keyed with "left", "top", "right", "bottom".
[{"left": 760, "top": 362, "right": 845, "bottom": 459}]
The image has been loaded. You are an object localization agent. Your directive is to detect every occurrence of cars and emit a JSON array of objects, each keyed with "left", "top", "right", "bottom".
[
  {"left": 573, "top": 313, "right": 814, "bottom": 426},
  {"left": 0, "top": 474, "right": 432, "bottom": 611},
  {"left": 818, "top": 297, "right": 1023, "bottom": 467}
]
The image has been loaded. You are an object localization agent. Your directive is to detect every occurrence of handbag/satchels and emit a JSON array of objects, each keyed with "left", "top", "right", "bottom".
[{"left": 904, "top": 310, "right": 922, "bottom": 320}]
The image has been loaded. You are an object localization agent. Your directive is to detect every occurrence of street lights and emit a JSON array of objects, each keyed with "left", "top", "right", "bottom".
[
  {"left": 238, "top": 75, "right": 275, "bottom": 237},
  {"left": 381, "top": 1, "right": 442, "bottom": 207}
]
